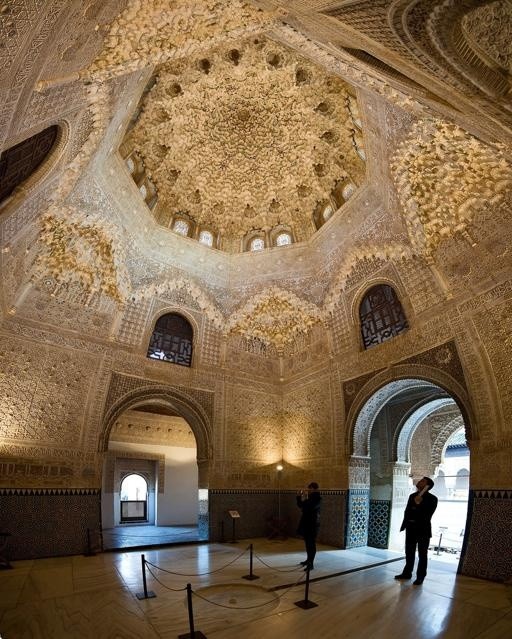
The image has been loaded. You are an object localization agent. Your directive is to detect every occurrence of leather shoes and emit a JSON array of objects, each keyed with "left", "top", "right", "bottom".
[
  {"left": 395, "top": 575, "right": 411, "bottom": 579},
  {"left": 413, "top": 579, "right": 423, "bottom": 585},
  {"left": 304, "top": 567, "right": 313, "bottom": 571},
  {"left": 301, "top": 561, "right": 307, "bottom": 565}
]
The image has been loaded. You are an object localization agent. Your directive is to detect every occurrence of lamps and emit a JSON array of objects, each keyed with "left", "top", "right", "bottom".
[{"left": 276, "top": 464, "right": 283, "bottom": 536}]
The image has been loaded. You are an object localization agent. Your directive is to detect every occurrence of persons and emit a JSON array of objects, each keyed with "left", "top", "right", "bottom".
[
  {"left": 296, "top": 482, "right": 321, "bottom": 571},
  {"left": 394, "top": 477, "right": 437, "bottom": 586}
]
